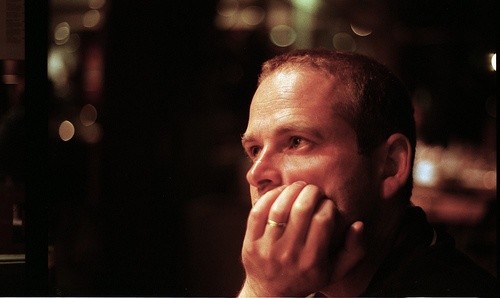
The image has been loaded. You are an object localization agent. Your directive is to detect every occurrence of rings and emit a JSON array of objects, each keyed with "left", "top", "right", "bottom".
[{"left": 267, "top": 220, "right": 287, "bottom": 230}]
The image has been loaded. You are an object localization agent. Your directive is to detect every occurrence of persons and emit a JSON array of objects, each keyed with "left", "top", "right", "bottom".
[{"left": 237, "top": 50, "right": 500, "bottom": 298}]
